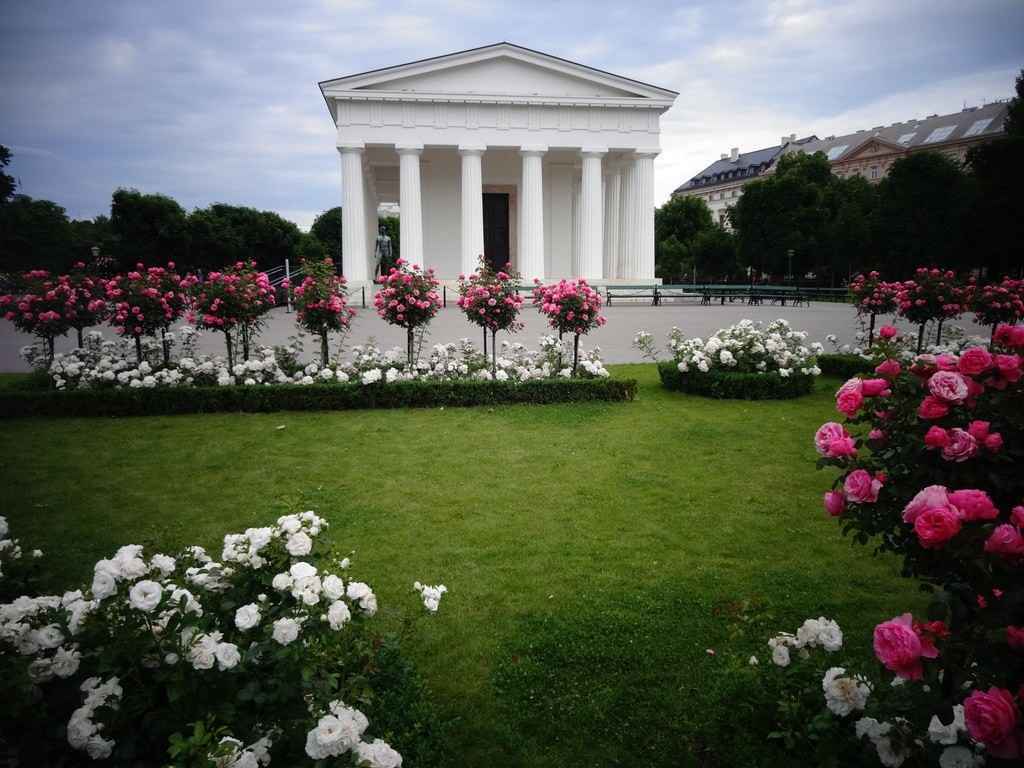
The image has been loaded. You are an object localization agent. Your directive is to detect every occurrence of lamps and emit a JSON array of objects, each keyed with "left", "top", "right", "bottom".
[
  {"left": 91, "top": 247, "right": 100, "bottom": 257},
  {"left": 787, "top": 250, "right": 794, "bottom": 258}
]
